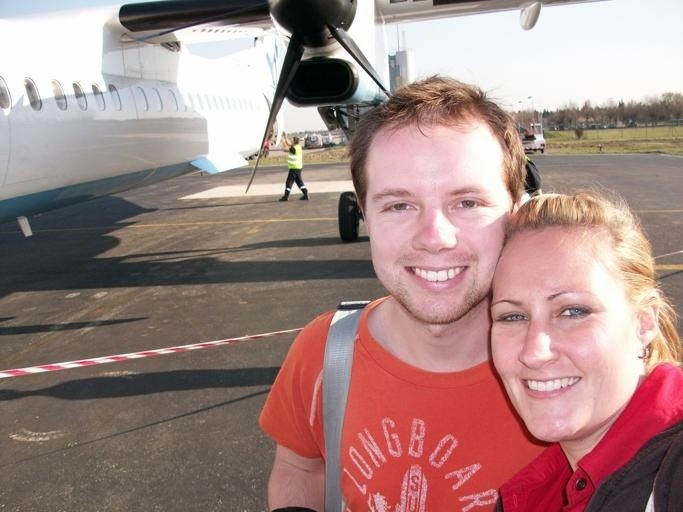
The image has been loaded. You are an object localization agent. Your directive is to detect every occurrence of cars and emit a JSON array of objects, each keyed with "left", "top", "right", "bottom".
[{"left": 566, "top": 121, "right": 637, "bottom": 128}]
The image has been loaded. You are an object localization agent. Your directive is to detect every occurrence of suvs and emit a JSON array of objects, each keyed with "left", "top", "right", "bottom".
[
  {"left": 516, "top": 123, "right": 546, "bottom": 154},
  {"left": 303, "top": 131, "right": 335, "bottom": 149}
]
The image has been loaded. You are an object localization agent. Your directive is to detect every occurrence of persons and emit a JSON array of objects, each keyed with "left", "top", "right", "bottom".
[
  {"left": 258, "top": 75, "right": 553, "bottom": 512},
  {"left": 524, "top": 155, "right": 543, "bottom": 198},
  {"left": 267, "top": 130, "right": 309, "bottom": 201},
  {"left": 491, "top": 184, "right": 683, "bottom": 512}
]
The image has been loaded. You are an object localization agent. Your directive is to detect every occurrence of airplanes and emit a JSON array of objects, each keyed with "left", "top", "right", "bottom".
[{"left": 0, "top": 0, "right": 605, "bottom": 244}]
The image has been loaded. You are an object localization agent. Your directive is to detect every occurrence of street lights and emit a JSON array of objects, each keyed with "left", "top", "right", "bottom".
[{"left": 508, "top": 95, "right": 534, "bottom": 122}]
